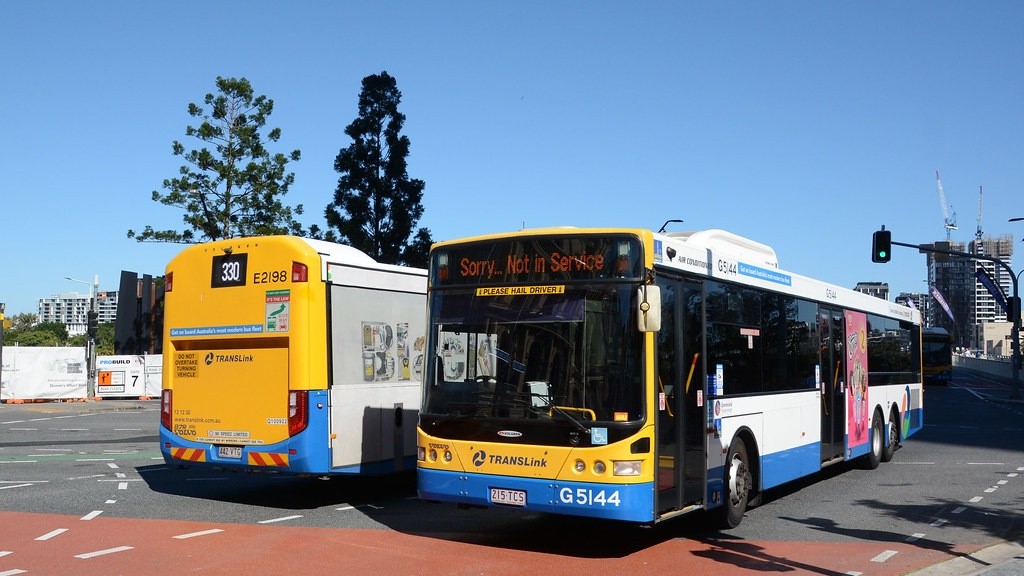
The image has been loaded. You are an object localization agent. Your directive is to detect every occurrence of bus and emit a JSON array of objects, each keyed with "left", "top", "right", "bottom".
[
  {"left": 918, "top": 326, "right": 954, "bottom": 387},
  {"left": 158, "top": 235, "right": 502, "bottom": 490},
  {"left": 415, "top": 229, "right": 925, "bottom": 536}
]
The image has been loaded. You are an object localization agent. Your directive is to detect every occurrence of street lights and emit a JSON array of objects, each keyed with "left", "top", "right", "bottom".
[{"left": 66, "top": 277, "right": 92, "bottom": 364}]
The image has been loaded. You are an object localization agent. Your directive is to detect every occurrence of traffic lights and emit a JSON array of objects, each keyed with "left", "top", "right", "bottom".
[
  {"left": 87, "top": 310, "right": 98, "bottom": 336},
  {"left": 873, "top": 231, "right": 892, "bottom": 262}
]
